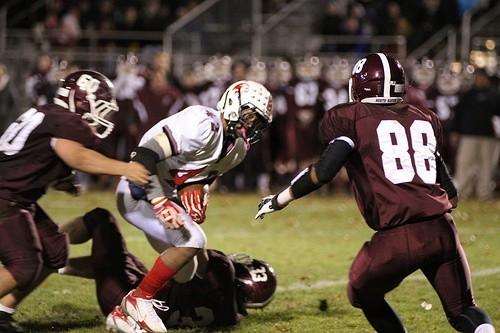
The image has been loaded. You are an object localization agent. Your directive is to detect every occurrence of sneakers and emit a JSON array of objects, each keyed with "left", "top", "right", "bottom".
[
  {"left": 105, "top": 305, "right": 147, "bottom": 333},
  {"left": 121, "top": 288, "right": 169, "bottom": 333}
]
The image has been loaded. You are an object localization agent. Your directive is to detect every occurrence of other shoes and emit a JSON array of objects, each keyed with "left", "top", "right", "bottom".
[{"left": 0, "top": 310, "right": 22, "bottom": 333}]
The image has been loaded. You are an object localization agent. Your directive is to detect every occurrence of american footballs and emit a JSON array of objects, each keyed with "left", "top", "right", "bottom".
[{"left": 177, "top": 183, "right": 206, "bottom": 224}]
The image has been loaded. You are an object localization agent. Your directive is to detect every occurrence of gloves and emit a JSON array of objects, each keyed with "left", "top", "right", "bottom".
[
  {"left": 151, "top": 195, "right": 184, "bottom": 229},
  {"left": 254, "top": 193, "right": 289, "bottom": 220}
]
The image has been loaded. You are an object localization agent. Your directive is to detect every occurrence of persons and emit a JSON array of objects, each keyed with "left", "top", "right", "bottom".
[
  {"left": 0, "top": 69, "right": 148, "bottom": 333},
  {"left": 112, "top": 53, "right": 352, "bottom": 197},
  {"left": 105, "top": 79, "right": 273, "bottom": 333},
  {"left": 57, "top": 208, "right": 276, "bottom": 330},
  {"left": 312, "top": 0, "right": 461, "bottom": 58},
  {"left": 403, "top": 48, "right": 500, "bottom": 204},
  {"left": 471, "top": 0, "right": 493, "bottom": 22},
  {"left": 6, "top": 0, "right": 201, "bottom": 55},
  {"left": 255, "top": 53, "right": 495, "bottom": 333},
  {"left": 0, "top": 53, "right": 87, "bottom": 192}
]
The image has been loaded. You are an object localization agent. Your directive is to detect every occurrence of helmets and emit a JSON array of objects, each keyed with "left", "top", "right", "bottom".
[
  {"left": 229, "top": 253, "right": 277, "bottom": 309},
  {"left": 216, "top": 80, "right": 273, "bottom": 123},
  {"left": 52, "top": 70, "right": 117, "bottom": 126},
  {"left": 349, "top": 52, "right": 405, "bottom": 104}
]
